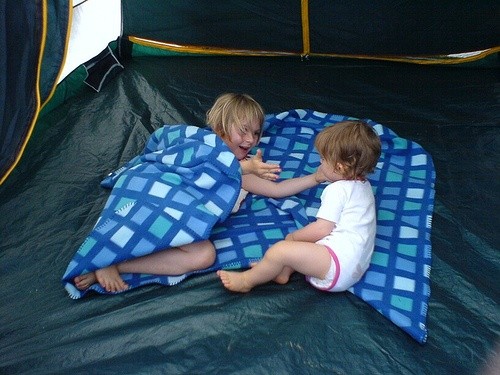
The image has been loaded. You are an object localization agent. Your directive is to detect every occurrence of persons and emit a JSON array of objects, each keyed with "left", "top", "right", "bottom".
[
  {"left": 217, "top": 121, "right": 381, "bottom": 292},
  {"left": 74, "top": 92, "right": 333, "bottom": 293}
]
